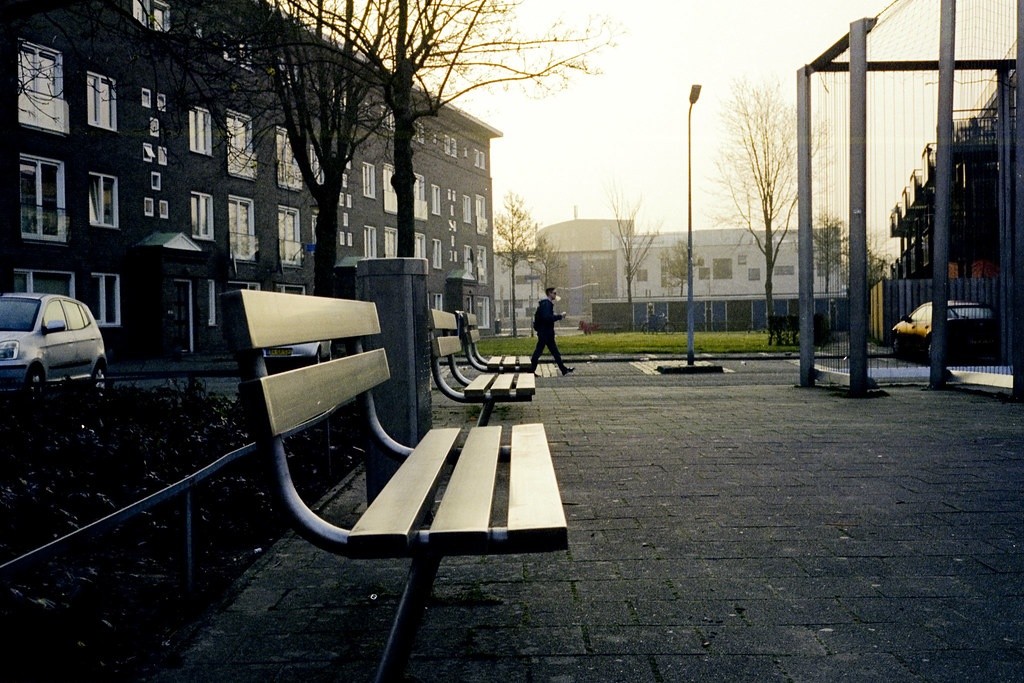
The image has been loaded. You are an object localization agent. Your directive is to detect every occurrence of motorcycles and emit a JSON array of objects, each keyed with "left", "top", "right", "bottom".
[{"left": 641, "top": 312, "right": 676, "bottom": 336}]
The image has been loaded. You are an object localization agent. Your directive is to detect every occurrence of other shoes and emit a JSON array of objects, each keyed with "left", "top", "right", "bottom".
[{"left": 562, "top": 366, "right": 575, "bottom": 376}]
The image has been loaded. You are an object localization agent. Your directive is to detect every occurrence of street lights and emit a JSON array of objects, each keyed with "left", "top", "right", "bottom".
[{"left": 687, "top": 84, "right": 701, "bottom": 366}]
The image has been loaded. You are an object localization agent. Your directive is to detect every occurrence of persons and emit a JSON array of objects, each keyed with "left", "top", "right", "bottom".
[{"left": 525, "top": 287, "right": 576, "bottom": 377}]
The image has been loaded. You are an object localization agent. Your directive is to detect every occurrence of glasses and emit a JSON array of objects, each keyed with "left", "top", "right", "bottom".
[{"left": 551, "top": 291, "right": 557, "bottom": 295}]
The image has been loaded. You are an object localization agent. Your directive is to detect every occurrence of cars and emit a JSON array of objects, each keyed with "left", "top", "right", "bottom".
[
  {"left": 890, "top": 301, "right": 1001, "bottom": 366},
  {"left": 264, "top": 339, "right": 332, "bottom": 364}
]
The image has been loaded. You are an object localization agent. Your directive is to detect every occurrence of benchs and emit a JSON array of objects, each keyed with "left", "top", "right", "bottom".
[
  {"left": 464, "top": 313, "right": 532, "bottom": 373},
  {"left": 427, "top": 307, "right": 536, "bottom": 427},
  {"left": 226, "top": 286, "right": 570, "bottom": 683}
]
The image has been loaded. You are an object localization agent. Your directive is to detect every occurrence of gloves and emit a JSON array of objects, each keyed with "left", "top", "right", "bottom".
[{"left": 560, "top": 311, "right": 567, "bottom": 320}]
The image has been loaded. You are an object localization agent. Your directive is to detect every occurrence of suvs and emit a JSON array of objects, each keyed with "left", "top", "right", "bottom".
[{"left": 0, "top": 292, "right": 108, "bottom": 413}]
